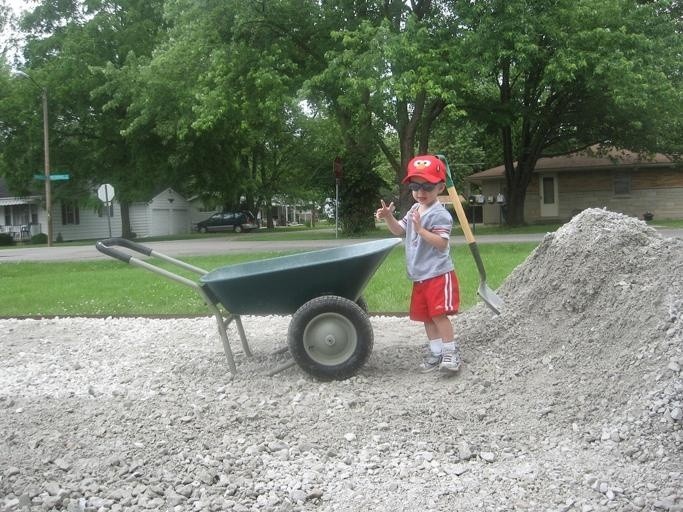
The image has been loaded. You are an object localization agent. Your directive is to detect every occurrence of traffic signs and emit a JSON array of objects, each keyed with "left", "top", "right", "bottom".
[
  {"left": 49, "top": 174, "right": 69, "bottom": 180},
  {"left": 35, "top": 174, "right": 47, "bottom": 180}
]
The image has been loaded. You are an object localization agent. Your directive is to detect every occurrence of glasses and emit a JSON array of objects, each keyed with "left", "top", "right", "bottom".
[{"left": 407, "top": 181, "right": 437, "bottom": 192}]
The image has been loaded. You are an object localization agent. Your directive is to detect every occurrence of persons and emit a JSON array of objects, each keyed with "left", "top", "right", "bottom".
[{"left": 375, "top": 157, "right": 460, "bottom": 373}]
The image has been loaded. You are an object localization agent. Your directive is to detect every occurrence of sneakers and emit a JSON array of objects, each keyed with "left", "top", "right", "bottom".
[
  {"left": 419, "top": 353, "right": 442, "bottom": 374},
  {"left": 439, "top": 348, "right": 462, "bottom": 373}
]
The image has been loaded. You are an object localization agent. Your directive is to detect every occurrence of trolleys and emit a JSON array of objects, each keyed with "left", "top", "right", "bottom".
[{"left": 95, "top": 237, "right": 403, "bottom": 381}]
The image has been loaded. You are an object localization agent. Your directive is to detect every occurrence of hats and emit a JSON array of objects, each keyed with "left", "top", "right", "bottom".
[{"left": 401, "top": 154, "right": 446, "bottom": 186}]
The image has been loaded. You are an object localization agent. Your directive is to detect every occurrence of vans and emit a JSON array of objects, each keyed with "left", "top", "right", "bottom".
[{"left": 192, "top": 210, "right": 258, "bottom": 233}]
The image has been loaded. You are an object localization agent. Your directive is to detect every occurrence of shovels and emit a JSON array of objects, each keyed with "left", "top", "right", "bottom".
[{"left": 436, "top": 155, "right": 506, "bottom": 315}]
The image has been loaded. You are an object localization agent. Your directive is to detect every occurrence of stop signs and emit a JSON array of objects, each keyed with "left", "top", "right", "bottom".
[{"left": 332, "top": 157, "right": 342, "bottom": 177}]
